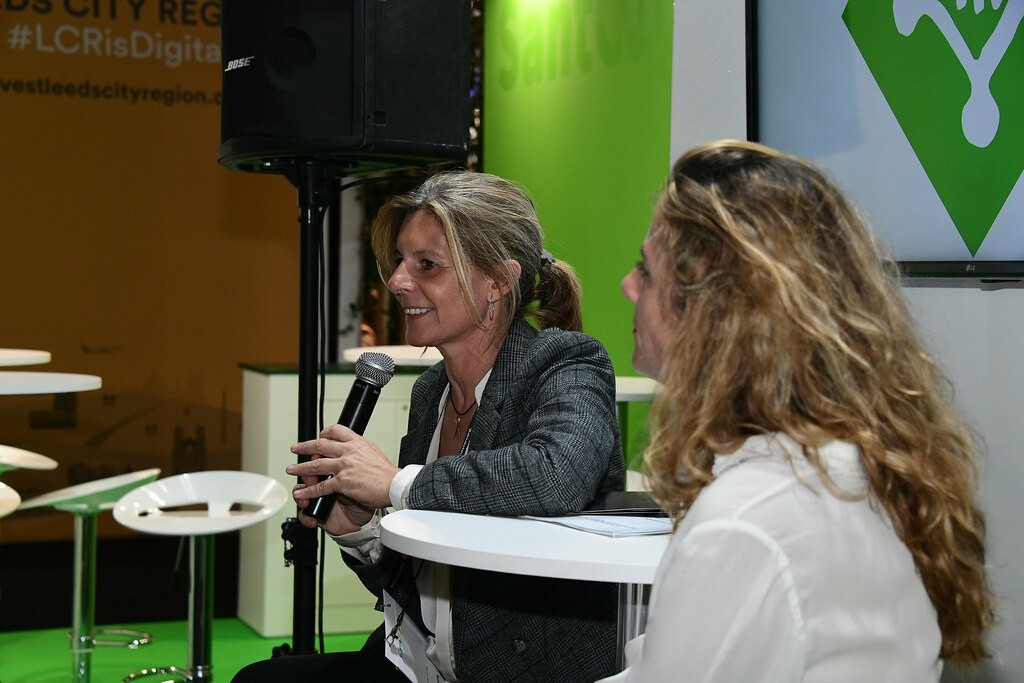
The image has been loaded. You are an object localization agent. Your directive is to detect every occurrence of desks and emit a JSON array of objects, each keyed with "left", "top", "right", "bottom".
[
  {"left": 380, "top": 508, "right": 679, "bottom": 673},
  {"left": 346, "top": 346, "right": 664, "bottom": 467},
  {"left": 0, "top": 371, "right": 101, "bottom": 395}
]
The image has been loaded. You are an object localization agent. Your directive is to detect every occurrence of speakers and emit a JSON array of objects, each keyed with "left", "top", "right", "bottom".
[{"left": 218, "top": 0, "right": 473, "bottom": 178}]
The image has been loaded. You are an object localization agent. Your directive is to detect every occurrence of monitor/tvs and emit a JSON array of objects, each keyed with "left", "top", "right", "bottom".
[{"left": 744, "top": 0, "right": 1024, "bottom": 281}]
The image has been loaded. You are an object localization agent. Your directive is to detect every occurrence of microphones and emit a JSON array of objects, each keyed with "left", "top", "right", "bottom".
[{"left": 303, "top": 351, "right": 396, "bottom": 526}]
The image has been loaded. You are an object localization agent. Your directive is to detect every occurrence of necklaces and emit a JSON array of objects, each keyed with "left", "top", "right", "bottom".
[{"left": 450, "top": 387, "right": 476, "bottom": 439}]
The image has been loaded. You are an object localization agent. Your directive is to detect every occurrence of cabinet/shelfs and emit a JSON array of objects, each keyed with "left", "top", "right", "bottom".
[{"left": 235, "top": 360, "right": 431, "bottom": 636}]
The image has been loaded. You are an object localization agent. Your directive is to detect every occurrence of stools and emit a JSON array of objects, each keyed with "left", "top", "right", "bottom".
[{"left": 0, "top": 442, "right": 288, "bottom": 683}]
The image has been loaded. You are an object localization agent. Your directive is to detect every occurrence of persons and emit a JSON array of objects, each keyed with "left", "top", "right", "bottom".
[
  {"left": 229, "top": 173, "right": 627, "bottom": 683},
  {"left": 591, "top": 137, "right": 997, "bottom": 683}
]
